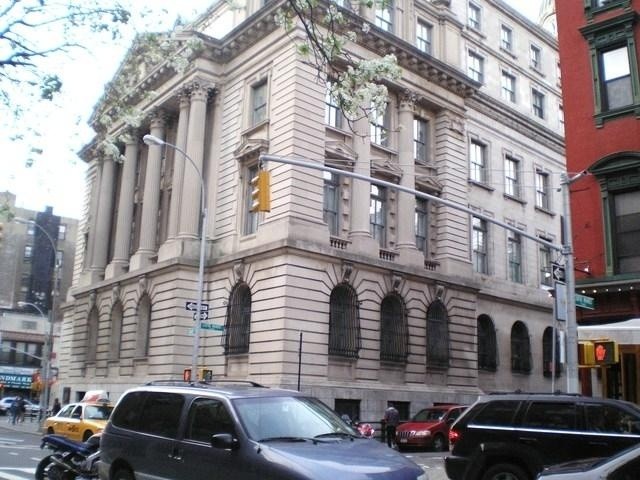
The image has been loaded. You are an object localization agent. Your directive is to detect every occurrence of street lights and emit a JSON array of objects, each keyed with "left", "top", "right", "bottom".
[
  {"left": 142, "top": 133, "right": 210, "bottom": 385},
  {"left": 16, "top": 298, "right": 51, "bottom": 428},
  {"left": 13, "top": 213, "right": 60, "bottom": 433}
]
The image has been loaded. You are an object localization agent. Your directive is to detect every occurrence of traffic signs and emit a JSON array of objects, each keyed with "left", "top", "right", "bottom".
[
  {"left": 185, "top": 300, "right": 209, "bottom": 311},
  {"left": 185, "top": 327, "right": 197, "bottom": 334},
  {"left": 193, "top": 311, "right": 208, "bottom": 320},
  {"left": 574, "top": 293, "right": 597, "bottom": 310},
  {"left": 552, "top": 263, "right": 567, "bottom": 285},
  {"left": 201, "top": 322, "right": 225, "bottom": 332}
]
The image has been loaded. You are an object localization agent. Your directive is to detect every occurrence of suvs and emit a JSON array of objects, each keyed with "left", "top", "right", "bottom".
[
  {"left": 442, "top": 390, "right": 640, "bottom": 480},
  {"left": 394, "top": 403, "right": 473, "bottom": 454},
  {"left": 1, "top": 395, "right": 42, "bottom": 418}
]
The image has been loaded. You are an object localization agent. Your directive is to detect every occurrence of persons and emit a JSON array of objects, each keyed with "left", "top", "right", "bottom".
[
  {"left": 8, "top": 396, "right": 18, "bottom": 425},
  {"left": 52, "top": 398, "right": 61, "bottom": 417},
  {"left": 17, "top": 396, "right": 25, "bottom": 424},
  {"left": 384, "top": 403, "right": 400, "bottom": 448}
]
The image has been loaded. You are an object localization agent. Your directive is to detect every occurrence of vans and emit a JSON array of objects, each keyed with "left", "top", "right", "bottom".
[{"left": 96, "top": 380, "right": 433, "bottom": 480}]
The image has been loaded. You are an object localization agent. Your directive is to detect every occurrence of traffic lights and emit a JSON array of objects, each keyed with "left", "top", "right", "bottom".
[
  {"left": 247, "top": 169, "right": 271, "bottom": 213},
  {"left": 198, "top": 367, "right": 214, "bottom": 384},
  {"left": 577, "top": 341, "right": 596, "bottom": 367},
  {"left": 184, "top": 369, "right": 191, "bottom": 381},
  {"left": 594, "top": 339, "right": 618, "bottom": 367},
  {"left": 31, "top": 373, "right": 42, "bottom": 393}
]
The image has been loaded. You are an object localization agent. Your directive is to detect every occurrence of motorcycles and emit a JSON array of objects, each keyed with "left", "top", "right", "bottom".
[{"left": 35, "top": 432, "right": 101, "bottom": 479}]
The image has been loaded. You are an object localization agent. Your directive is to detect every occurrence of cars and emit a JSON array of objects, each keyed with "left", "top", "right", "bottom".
[
  {"left": 535, "top": 440, "right": 640, "bottom": 480},
  {"left": 43, "top": 388, "right": 117, "bottom": 443}
]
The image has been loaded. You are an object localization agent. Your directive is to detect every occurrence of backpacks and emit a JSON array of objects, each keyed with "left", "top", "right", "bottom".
[{"left": 389, "top": 410, "right": 398, "bottom": 428}]
